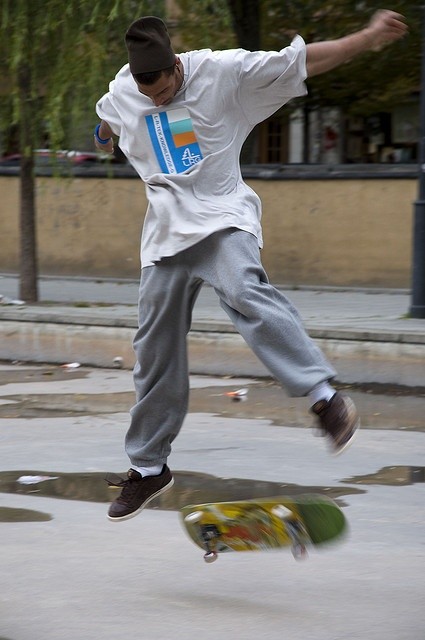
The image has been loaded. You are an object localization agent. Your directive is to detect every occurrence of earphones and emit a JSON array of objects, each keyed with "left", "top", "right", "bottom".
[{"left": 173, "top": 62, "right": 182, "bottom": 70}]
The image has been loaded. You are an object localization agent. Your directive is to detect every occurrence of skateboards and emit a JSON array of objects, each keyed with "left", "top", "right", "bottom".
[{"left": 179, "top": 491, "right": 347, "bottom": 564}]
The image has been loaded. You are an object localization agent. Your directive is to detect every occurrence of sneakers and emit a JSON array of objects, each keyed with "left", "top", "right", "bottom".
[
  {"left": 311, "top": 392, "right": 358, "bottom": 445},
  {"left": 104, "top": 464, "right": 174, "bottom": 523}
]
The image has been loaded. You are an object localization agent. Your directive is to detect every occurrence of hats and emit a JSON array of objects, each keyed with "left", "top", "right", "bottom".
[{"left": 124, "top": 16, "right": 177, "bottom": 75}]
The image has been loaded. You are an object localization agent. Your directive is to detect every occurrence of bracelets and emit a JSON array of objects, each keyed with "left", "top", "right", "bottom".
[{"left": 94, "top": 125, "right": 111, "bottom": 144}]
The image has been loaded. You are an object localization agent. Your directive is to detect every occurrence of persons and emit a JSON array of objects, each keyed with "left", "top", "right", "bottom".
[{"left": 93, "top": 9, "right": 409, "bottom": 522}]
